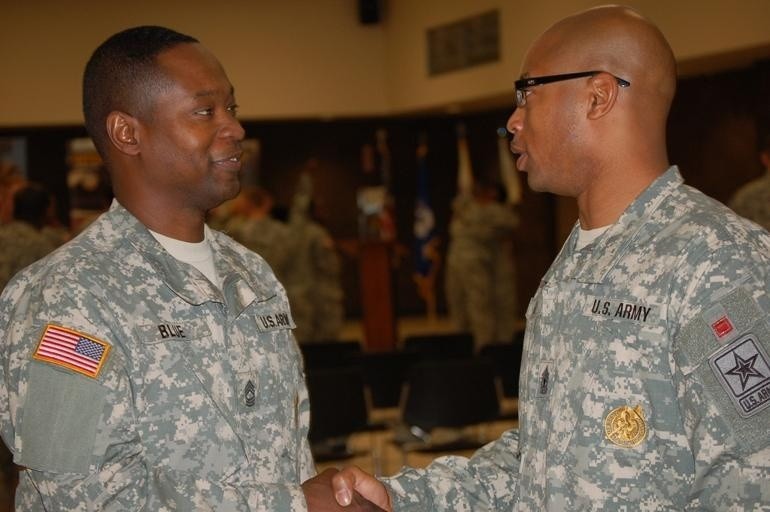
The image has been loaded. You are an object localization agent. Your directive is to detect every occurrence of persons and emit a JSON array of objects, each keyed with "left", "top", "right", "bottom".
[
  {"left": 727, "top": 124, "right": 770, "bottom": 230},
  {"left": 207, "top": 182, "right": 521, "bottom": 344},
  {"left": 0, "top": 23, "right": 388, "bottom": 511},
  {"left": 1, "top": 184, "right": 65, "bottom": 289},
  {"left": 330, "top": 1, "right": 770, "bottom": 511}
]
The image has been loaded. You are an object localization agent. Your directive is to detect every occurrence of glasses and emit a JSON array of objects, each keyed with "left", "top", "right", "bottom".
[{"left": 513, "top": 69, "right": 631, "bottom": 108}]
